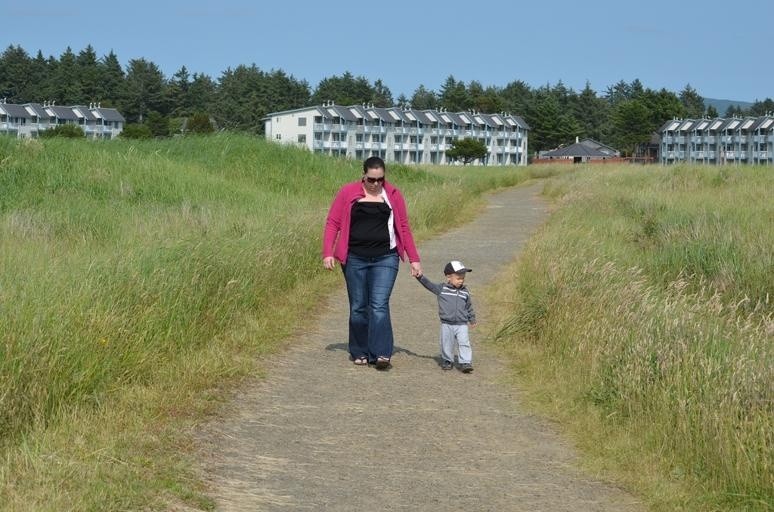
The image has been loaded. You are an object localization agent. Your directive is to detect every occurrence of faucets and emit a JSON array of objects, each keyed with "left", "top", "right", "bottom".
[
  {"left": 354, "top": 355, "right": 368, "bottom": 364},
  {"left": 376, "top": 355, "right": 390, "bottom": 369}
]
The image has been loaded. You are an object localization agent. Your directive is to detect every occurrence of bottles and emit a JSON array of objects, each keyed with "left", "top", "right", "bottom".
[{"left": 444, "top": 261, "right": 472, "bottom": 276}]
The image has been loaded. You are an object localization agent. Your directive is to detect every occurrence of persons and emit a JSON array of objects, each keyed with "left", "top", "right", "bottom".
[
  {"left": 409, "top": 259, "right": 477, "bottom": 374},
  {"left": 321, "top": 157, "right": 424, "bottom": 369}
]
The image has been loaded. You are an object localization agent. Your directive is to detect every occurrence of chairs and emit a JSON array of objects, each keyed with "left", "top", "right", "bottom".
[
  {"left": 462, "top": 364, "right": 474, "bottom": 373},
  {"left": 442, "top": 359, "right": 452, "bottom": 370}
]
